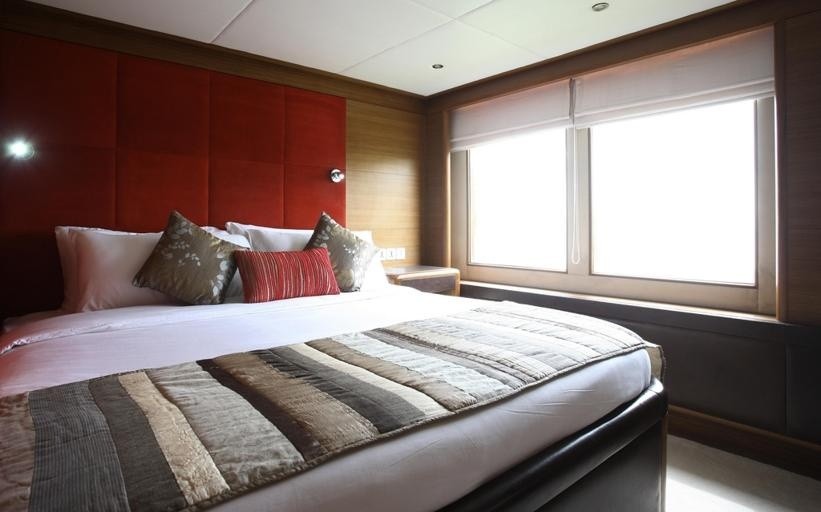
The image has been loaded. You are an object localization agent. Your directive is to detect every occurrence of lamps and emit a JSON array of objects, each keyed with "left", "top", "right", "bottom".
[{"left": 331, "top": 168, "right": 344, "bottom": 182}]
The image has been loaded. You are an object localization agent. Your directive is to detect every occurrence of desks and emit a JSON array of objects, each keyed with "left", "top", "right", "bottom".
[{"left": 385, "top": 264, "right": 461, "bottom": 297}]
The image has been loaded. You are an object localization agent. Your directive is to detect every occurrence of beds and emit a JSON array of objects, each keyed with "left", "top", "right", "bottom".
[{"left": 0, "top": 285, "right": 666, "bottom": 512}]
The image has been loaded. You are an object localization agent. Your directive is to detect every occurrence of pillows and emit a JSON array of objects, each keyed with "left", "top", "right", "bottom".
[
  {"left": 246, "top": 227, "right": 388, "bottom": 287},
  {"left": 226, "top": 221, "right": 258, "bottom": 238},
  {"left": 132, "top": 211, "right": 249, "bottom": 305},
  {"left": 304, "top": 213, "right": 377, "bottom": 292},
  {"left": 68, "top": 228, "right": 164, "bottom": 311},
  {"left": 232, "top": 249, "right": 341, "bottom": 301},
  {"left": 55, "top": 226, "right": 139, "bottom": 313}
]
